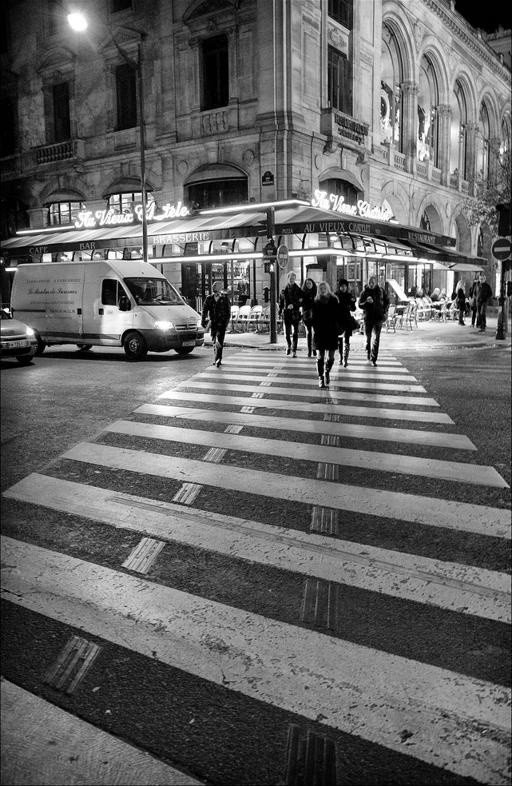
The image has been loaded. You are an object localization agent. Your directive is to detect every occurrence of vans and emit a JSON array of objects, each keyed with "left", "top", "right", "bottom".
[{"left": 8, "top": 259, "right": 205, "bottom": 359}]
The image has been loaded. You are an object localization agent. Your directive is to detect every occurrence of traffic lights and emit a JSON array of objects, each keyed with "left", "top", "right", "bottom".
[{"left": 257, "top": 206, "right": 275, "bottom": 237}]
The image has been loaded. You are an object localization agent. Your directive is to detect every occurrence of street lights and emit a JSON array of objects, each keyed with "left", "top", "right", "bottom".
[{"left": 65, "top": 7, "right": 149, "bottom": 261}]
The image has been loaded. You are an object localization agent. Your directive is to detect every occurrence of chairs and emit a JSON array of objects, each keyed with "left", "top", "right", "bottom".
[{"left": 225, "top": 294, "right": 460, "bottom": 336}]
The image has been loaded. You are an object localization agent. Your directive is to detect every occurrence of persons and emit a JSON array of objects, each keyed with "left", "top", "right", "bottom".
[
  {"left": 298, "top": 278, "right": 318, "bottom": 360},
  {"left": 276, "top": 271, "right": 301, "bottom": 358},
  {"left": 199, "top": 281, "right": 231, "bottom": 369},
  {"left": 334, "top": 279, "right": 358, "bottom": 369},
  {"left": 312, "top": 280, "right": 341, "bottom": 389},
  {"left": 357, "top": 275, "right": 389, "bottom": 368},
  {"left": 430, "top": 274, "right": 493, "bottom": 333}
]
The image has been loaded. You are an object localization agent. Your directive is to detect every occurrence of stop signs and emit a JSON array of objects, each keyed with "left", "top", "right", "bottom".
[
  {"left": 492, "top": 238, "right": 512, "bottom": 262},
  {"left": 277, "top": 244, "right": 290, "bottom": 269}
]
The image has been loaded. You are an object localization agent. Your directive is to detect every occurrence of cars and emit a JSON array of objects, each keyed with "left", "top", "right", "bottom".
[{"left": 1, "top": 306, "right": 37, "bottom": 365}]
